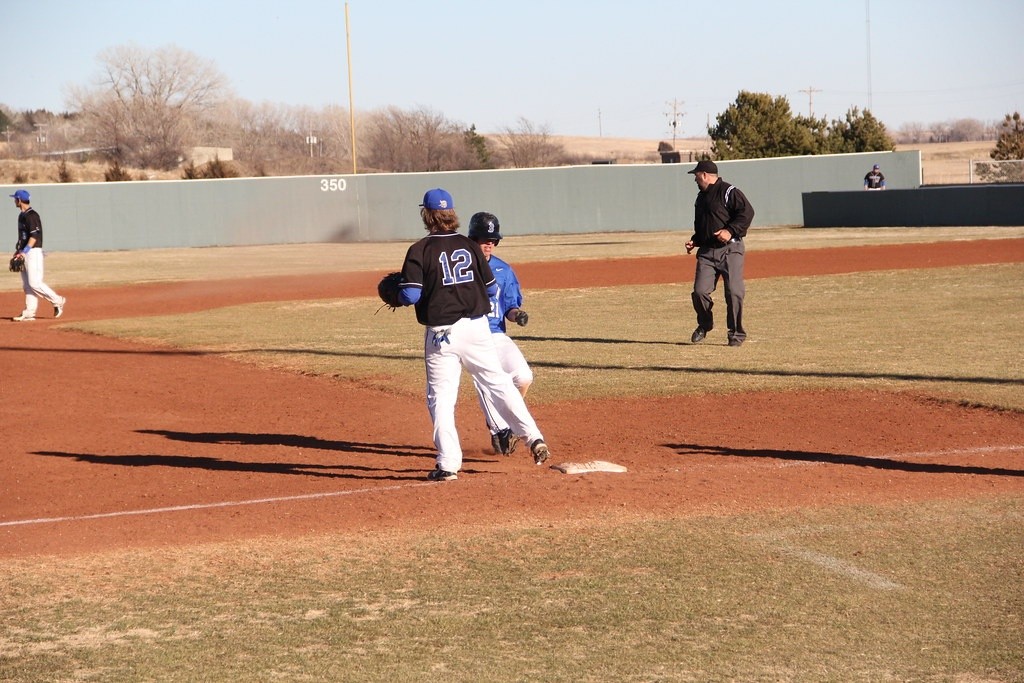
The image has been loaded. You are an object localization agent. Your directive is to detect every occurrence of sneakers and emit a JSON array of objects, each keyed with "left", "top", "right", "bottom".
[
  {"left": 53, "top": 296, "right": 66, "bottom": 317},
  {"left": 497, "top": 428, "right": 517, "bottom": 456},
  {"left": 12, "top": 310, "right": 36, "bottom": 321},
  {"left": 491, "top": 435, "right": 503, "bottom": 454},
  {"left": 531, "top": 439, "right": 548, "bottom": 464},
  {"left": 426, "top": 464, "right": 458, "bottom": 480}
]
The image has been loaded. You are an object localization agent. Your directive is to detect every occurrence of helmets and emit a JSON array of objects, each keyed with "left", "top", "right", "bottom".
[
  {"left": 873, "top": 164, "right": 880, "bottom": 169},
  {"left": 468, "top": 212, "right": 502, "bottom": 246}
]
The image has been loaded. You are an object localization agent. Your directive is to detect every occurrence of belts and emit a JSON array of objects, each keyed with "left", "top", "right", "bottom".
[{"left": 705, "top": 237, "right": 739, "bottom": 248}]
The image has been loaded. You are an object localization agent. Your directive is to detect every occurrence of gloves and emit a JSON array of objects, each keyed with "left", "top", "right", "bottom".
[{"left": 515, "top": 310, "right": 528, "bottom": 326}]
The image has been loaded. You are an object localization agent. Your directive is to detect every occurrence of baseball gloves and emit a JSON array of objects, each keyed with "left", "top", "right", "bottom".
[
  {"left": 9, "top": 254, "right": 25, "bottom": 272},
  {"left": 377, "top": 272, "right": 404, "bottom": 307}
]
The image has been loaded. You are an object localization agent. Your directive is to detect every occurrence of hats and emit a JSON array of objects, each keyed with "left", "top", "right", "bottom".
[
  {"left": 418, "top": 188, "right": 453, "bottom": 210},
  {"left": 9, "top": 190, "right": 30, "bottom": 202},
  {"left": 688, "top": 161, "right": 718, "bottom": 173}
]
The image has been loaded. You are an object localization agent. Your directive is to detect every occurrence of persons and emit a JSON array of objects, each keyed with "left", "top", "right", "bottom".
[
  {"left": 467, "top": 212, "right": 533, "bottom": 456},
  {"left": 685, "top": 161, "right": 755, "bottom": 346},
  {"left": 377, "top": 189, "right": 549, "bottom": 480},
  {"left": 863, "top": 164, "right": 886, "bottom": 190},
  {"left": 9, "top": 190, "right": 66, "bottom": 321}
]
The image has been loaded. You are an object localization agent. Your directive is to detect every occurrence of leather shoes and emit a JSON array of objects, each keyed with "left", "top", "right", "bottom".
[
  {"left": 728, "top": 340, "right": 741, "bottom": 346},
  {"left": 691, "top": 325, "right": 713, "bottom": 342}
]
